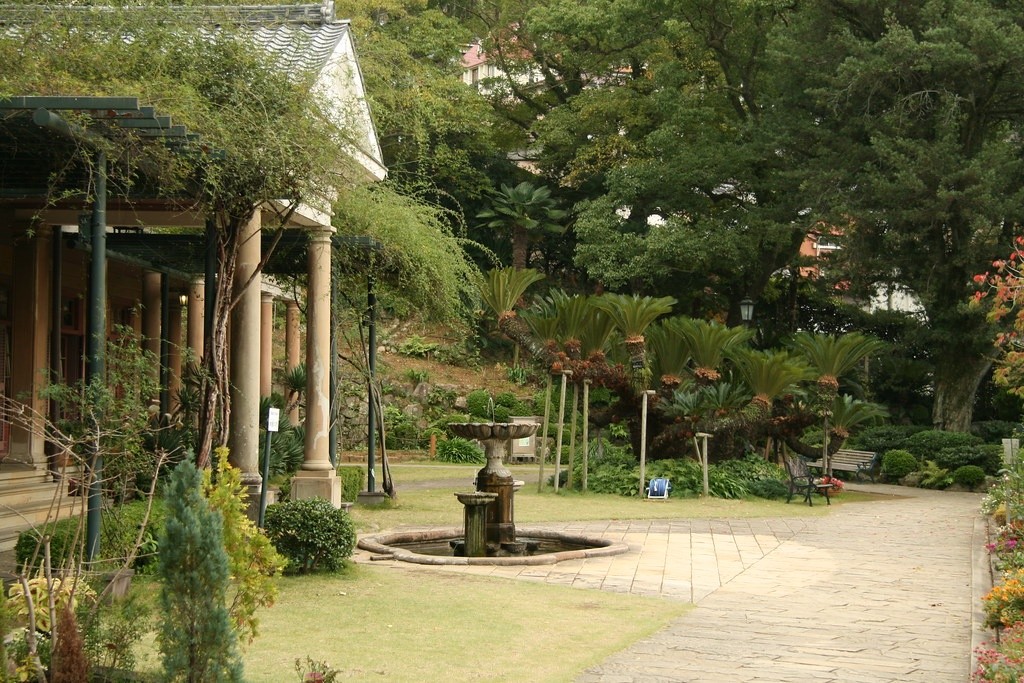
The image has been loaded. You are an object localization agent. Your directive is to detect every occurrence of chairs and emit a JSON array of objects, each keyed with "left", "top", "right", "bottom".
[{"left": 784, "top": 455, "right": 834, "bottom": 506}]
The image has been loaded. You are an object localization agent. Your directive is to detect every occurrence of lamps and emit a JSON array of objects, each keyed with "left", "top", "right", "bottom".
[{"left": 178, "top": 280, "right": 189, "bottom": 307}]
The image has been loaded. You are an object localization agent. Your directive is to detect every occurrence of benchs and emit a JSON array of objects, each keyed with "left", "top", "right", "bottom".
[{"left": 806, "top": 449, "right": 877, "bottom": 485}]
